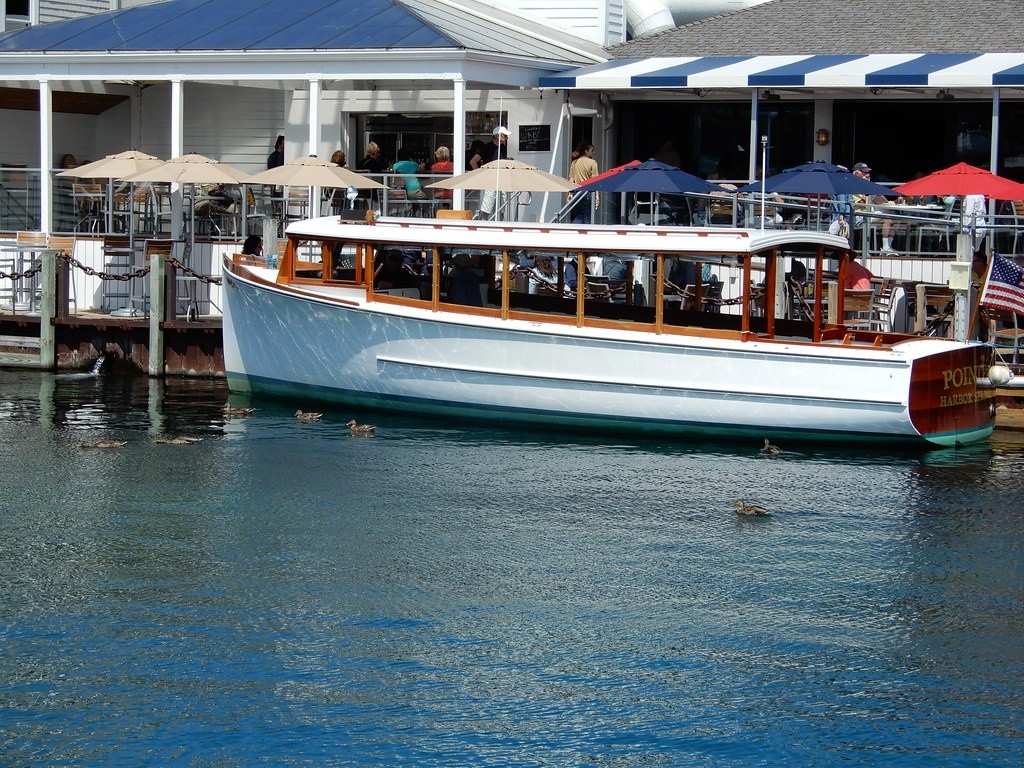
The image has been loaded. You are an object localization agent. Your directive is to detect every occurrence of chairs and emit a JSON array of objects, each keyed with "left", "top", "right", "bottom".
[
  {"left": 750, "top": 277, "right": 1024, "bottom": 365},
  {"left": 0, "top": 162, "right": 453, "bottom": 236},
  {"left": 708, "top": 195, "right": 744, "bottom": 224},
  {"left": 680, "top": 283, "right": 711, "bottom": 312},
  {"left": 587, "top": 281, "right": 614, "bottom": 303},
  {"left": 755, "top": 197, "right": 783, "bottom": 216},
  {"left": 1009, "top": 200, "right": 1024, "bottom": 254}
]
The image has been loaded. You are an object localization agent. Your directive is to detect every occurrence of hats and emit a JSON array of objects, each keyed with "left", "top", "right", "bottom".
[
  {"left": 853, "top": 162, "right": 872, "bottom": 173},
  {"left": 492, "top": 126, "right": 512, "bottom": 135}
]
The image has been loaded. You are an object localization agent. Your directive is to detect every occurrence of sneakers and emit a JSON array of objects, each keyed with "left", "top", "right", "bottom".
[{"left": 880, "top": 249, "right": 900, "bottom": 257}]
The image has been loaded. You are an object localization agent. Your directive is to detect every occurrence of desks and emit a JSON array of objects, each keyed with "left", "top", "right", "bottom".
[
  {"left": 0, "top": 246, "right": 66, "bottom": 316},
  {"left": 69, "top": 192, "right": 107, "bottom": 237},
  {"left": 194, "top": 195, "right": 228, "bottom": 242},
  {"left": 270, "top": 196, "right": 309, "bottom": 222}
]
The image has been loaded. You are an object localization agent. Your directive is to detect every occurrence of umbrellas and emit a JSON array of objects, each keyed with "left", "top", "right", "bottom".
[
  {"left": 892, "top": 163, "right": 1024, "bottom": 234},
  {"left": 56, "top": 148, "right": 166, "bottom": 308},
  {"left": 113, "top": 152, "right": 251, "bottom": 313},
  {"left": 239, "top": 154, "right": 390, "bottom": 262},
  {"left": 424, "top": 157, "right": 581, "bottom": 222},
  {"left": 570, "top": 158, "right": 729, "bottom": 277},
  {"left": 724, "top": 160, "right": 908, "bottom": 283}
]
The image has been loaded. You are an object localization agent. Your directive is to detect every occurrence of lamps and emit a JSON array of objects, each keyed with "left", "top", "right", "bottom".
[
  {"left": 760, "top": 89, "right": 780, "bottom": 100},
  {"left": 936, "top": 90, "right": 954, "bottom": 101},
  {"left": 816, "top": 128, "right": 829, "bottom": 146}
]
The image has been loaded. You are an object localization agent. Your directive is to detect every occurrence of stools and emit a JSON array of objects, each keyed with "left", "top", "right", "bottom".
[
  {"left": 100, "top": 235, "right": 136, "bottom": 315},
  {"left": 45, "top": 236, "right": 78, "bottom": 315},
  {"left": 13, "top": 229, "right": 47, "bottom": 299},
  {"left": 141, "top": 238, "right": 175, "bottom": 319}
]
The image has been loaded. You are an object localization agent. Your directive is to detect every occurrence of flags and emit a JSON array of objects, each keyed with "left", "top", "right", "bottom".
[{"left": 978, "top": 251, "right": 1024, "bottom": 316}]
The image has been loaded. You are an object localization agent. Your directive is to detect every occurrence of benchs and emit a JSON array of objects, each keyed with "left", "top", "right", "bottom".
[{"left": 774, "top": 223, "right": 1011, "bottom": 252}]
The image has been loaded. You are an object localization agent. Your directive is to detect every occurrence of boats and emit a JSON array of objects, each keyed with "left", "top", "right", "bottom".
[{"left": 220, "top": 92, "right": 997, "bottom": 450}]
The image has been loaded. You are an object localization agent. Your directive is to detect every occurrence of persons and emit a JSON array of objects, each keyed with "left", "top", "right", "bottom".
[
  {"left": 431, "top": 147, "right": 454, "bottom": 217},
  {"left": 356, "top": 142, "right": 386, "bottom": 204},
  {"left": 697, "top": 164, "right": 986, "bottom": 258},
  {"left": 373, "top": 248, "right": 722, "bottom": 313},
  {"left": 265, "top": 135, "right": 284, "bottom": 214},
  {"left": 241, "top": 235, "right": 263, "bottom": 257},
  {"left": 393, "top": 149, "right": 426, "bottom": 218},
  {"left": 567, "top": 144, "right": 600, "bottom": 224},
  {"left": 470, "top": 126, "right": 514, "bottom": 221},
  {"left": 325, "top": 150, "right": 352, "bottom": 214}
]
